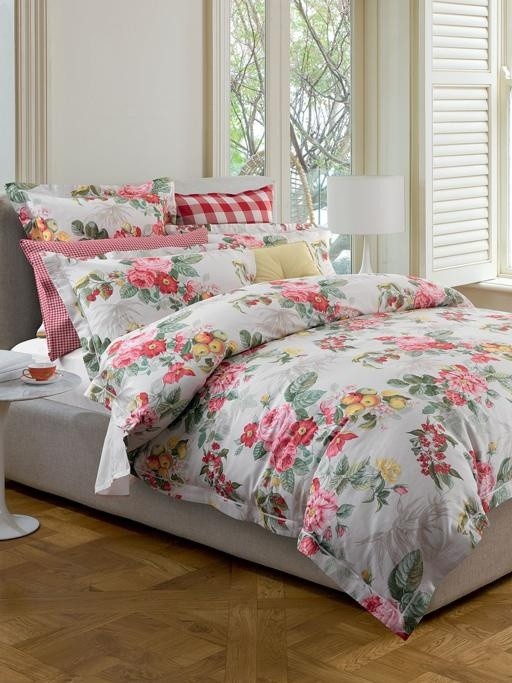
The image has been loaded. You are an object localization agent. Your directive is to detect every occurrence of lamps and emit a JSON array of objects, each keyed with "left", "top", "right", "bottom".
[{"left": 327, "top": 174, "right": 404, "bottom": 276}]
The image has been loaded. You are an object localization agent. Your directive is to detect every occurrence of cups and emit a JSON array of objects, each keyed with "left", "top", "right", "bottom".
[{"left": 23, "top": 362, "right": 56, "bottom": 380}]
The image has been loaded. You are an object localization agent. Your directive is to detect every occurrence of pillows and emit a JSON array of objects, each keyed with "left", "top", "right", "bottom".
[
  {"left": 164, "top": 222, "right": 334, "bottom": 281},
  {"left": 5, "top": 177, "right": 179, "bottom": 243},
  {"left": 20, "top": 227, "right": 210, "bottom": 363},
  {"left": 40, "top": 241, "right": 255, "bottom": 381},
  {"left": 178, "top": 185, "right": 275, "bottom": 227}
]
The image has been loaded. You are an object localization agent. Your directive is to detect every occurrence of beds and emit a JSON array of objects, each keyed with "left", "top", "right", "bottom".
[{"left": 1, "top": 184, "right": 512, "bottom": 618}]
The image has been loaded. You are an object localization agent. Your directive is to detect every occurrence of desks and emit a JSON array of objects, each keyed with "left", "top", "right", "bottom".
[{"left": 1, "top": 368, "right": 77, "bottom": 542}]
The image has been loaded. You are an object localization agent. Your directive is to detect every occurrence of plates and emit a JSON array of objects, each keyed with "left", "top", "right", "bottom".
[{"left": 19, "top": 371, "right": 65, "bottom": 384}]
